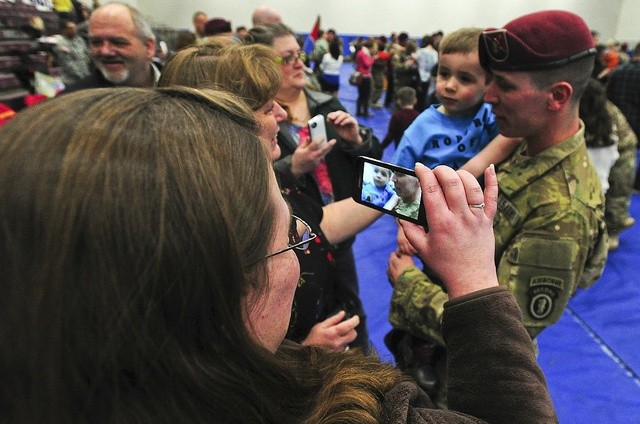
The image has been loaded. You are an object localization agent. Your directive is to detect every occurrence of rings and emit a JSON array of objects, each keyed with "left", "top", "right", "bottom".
[{"left": 469, "top": 202, "right": 485, "bottom": 208}]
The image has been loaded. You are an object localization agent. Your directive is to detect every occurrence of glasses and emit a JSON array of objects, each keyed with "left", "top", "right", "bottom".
[
  {"left": 248, "top": 216, "right": 317, "bottom": 266},
  {"left": 274, "top": 51, "right": 307, "bottom": 65}
]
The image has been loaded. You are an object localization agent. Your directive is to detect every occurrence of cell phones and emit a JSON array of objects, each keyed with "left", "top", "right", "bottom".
[
  {"left": 309, "top": 115, "right": 328, "bottom": 151},
  {"left": 352, "top": 155, "right": 426, "bottom": 227}
]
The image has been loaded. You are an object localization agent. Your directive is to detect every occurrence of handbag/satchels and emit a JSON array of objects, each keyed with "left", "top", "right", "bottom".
[{"left": 350, "top": 69, "right": 363, "bottom": 85}]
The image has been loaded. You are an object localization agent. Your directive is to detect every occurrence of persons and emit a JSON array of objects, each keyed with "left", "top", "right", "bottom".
[
  {"left": 390, "top": 30, "right": 415, "bottom": 100},
  {"left": 207, "top": 18, "right": 231, "bottom": 35},
  {"left": 588, "top": 28, "right": 630, "bottom": 79},
  {"left": 411, "top": 34, "right": 439, "bottom": 110},
  {"left": 361, "top": 161, "right": 401, "bottom": 211},
  {"left": 387, "top": 7, "right": 611, "bottom": 408},
  {"left": 232, "top": 25, "right": 248, "bottom": 41},
  {"left": 252, "top": 8, "right": 283, "bottom": 28},
  {"left": 59, "top": 2, "right": 167, "bottom": 92},
  {"left": 586, "top": 94, "right": 640, "bottom": 252},
  {"left": 52, "top": 20, "right": 94, "bottom": 81},
  {"left": 324, "top": 28, "right": 337, "bottom": 40},
  {"left": 318, "top": 36, "right": 344, "bottom": 98},
  {"left": 240, "top": 20, "right": 385, "bottom": 296},
  {"left": 154, "top": 32, "right": 384, "bottom": 352},
  {"left": 310, "top": 29, "right": 330, "bottom": 71},
  {"left": 351, "top": 40, "right": 382, "bottom": 117},
  {"left": 603, "top": 41, "right": 639, "bottom": 228},
  {"left": 189, "top": 11, "right": 210, "bottom": 34},
  {"left": 0, "top": 82, "right": 559, "bottom": 424},
  {"left": 378, "top": 83, "right": 422, "bottom": 151},
  {"left": 390, "top": 170, "right": 424, "bottom": 220},
  {"left": 368, "top": 36, "right": 387, "bottom": 109},
  {"left": 572, "top": 74, "right": 622, "bottom": 202}
]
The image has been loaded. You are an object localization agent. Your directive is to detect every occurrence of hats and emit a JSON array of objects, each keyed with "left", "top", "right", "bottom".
[{"left": 478, "top": 10, "right": 597, "bottom": 69}]
